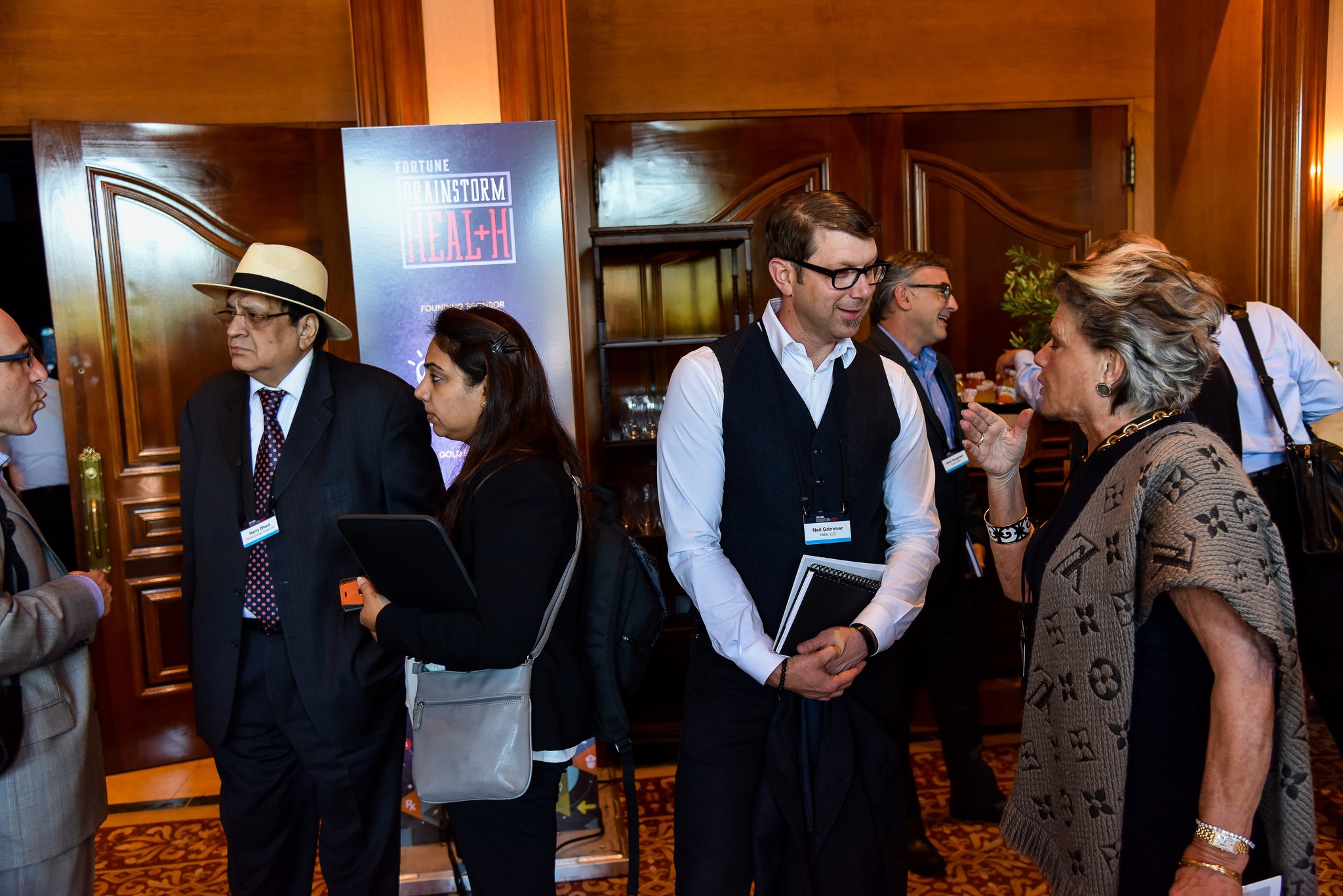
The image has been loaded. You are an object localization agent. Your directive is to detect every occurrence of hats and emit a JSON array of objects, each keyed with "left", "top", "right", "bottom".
[{"left": 192, "top": 242, "right": 352, "bottom": 341}]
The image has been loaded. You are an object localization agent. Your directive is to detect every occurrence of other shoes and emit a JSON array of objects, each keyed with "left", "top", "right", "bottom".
[
  {"left": 949, "top": 784, "right": 1008, "bottom": 823},
  {"left": 907, "top": 837, "right": 948, "bottom": 878}
]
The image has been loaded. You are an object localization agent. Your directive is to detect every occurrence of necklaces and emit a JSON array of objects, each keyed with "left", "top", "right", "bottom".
[{"left": 1082, "top": 409, "right": 1181, "bottom": 462}]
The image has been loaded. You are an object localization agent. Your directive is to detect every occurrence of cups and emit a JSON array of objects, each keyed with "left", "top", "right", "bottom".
[
  {"left": 619, "top": 393, "right": 670, "bottom": 439},
  {"left": 615, "top": 482, "right": 666, "bottom": 537}
]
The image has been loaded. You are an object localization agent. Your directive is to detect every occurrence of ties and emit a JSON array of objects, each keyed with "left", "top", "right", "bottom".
[{"left": 243, "top": 388, "right": 290, "bottom": 631}]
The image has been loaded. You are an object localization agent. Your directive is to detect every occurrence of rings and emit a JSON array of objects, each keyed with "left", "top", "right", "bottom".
[{"left": 970, "top": 432, "right": 984, "bottom": 447}]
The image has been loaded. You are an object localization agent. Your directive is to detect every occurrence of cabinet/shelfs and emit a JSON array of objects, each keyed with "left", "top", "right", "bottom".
[{"left": 589, "top": 220, "right": 752, "bottom": 762}]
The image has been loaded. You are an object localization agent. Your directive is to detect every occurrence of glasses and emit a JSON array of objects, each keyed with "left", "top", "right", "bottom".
[
  {"left": 908, "top": 284, "right": 954, "bottom": 303},
  {"left": 213, "top": 310, "right": 289, "bottom": 329},
  {"left": 781, "top": 256, "right": 891, "bottom": 290},
  {"left": 0, "top": 347, "right": 34, "bottom": 368}
]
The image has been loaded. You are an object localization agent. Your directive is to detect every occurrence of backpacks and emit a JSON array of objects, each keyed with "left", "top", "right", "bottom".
[{"left": 460, "top": 450, "right": 674, "bottom": 766}]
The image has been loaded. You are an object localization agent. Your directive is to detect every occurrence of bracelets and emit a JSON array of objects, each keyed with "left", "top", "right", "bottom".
[
  {"left": 1196, "top": 824, "right": 1249, "bottom": 855},
  {"left": 1195, "top": 818, "right": 1255, "bottom": 849},
  {"left": 848, "top": 623, "right": 875, "bottom": 661},
  {"left": 775, "top": 658, "right": 787, "bottom": 695},
  {"left": 984, "top": 506, "right": 1032, "bottom": 545},
  {"left": 1179, "top": 858, "right": 1242, "bottom": 886}
]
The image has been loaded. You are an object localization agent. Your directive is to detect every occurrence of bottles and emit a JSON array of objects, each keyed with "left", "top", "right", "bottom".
[{"left": 955, "top": 368, "right": 1027, "bottom": 406}]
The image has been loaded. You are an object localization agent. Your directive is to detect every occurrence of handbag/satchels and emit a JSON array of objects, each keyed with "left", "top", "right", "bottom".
[
  {"left": 1283, "top": 434, "right": 1343, "bottom": 555},
  {"left": 412, "top": 664, "right": 533, "bottom": 803},
  {"left": 0, "top": 496, "right": 30, "bottom": 776}
]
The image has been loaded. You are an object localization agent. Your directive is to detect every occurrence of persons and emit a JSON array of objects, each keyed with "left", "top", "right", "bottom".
[
  {"left": 358, "top": 306, "right": 597, "bottom": 896},
  {"left": 0, "top": 307, "right": 113, "bottom": 896},
  {"left": 861, "top": 250, "right": 1009, "bottom": 879},
  {"left": 959, "top": 230, "right": 1342, "bottom": 896},
  {"left": 181, "top": 242, "right": 450, "bottom": 896},
  {"left": 655, "top": 189, "right": 942, "bottom": 896}
]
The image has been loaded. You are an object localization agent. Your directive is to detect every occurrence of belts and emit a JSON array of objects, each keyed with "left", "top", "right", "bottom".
[{"left": 242, "top": 617, "right": 283, "bottom": 636}]
